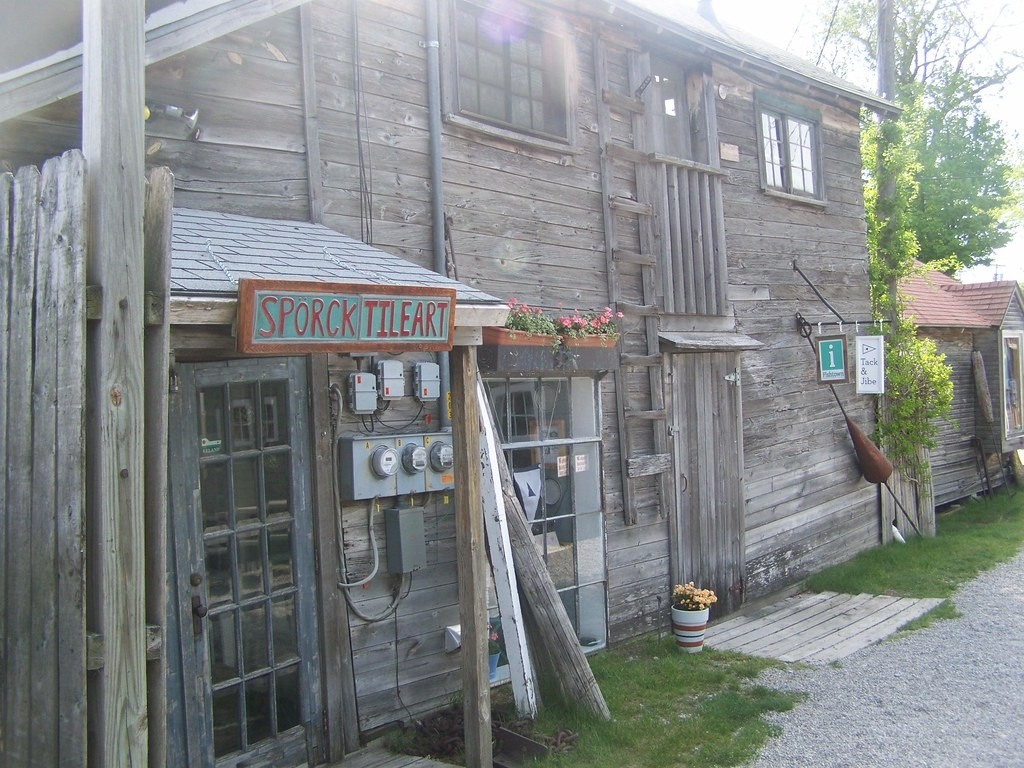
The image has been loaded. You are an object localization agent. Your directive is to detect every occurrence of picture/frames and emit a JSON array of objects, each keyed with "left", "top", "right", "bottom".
[
  {"left": 855, "top": 336, "right": 884, "bottom": 394},
  {"left": 814, "top": 334, "right": 849, "bottom": 385},
  {"left": 529, "top": 419, "right": 567, "bottom": 471}
]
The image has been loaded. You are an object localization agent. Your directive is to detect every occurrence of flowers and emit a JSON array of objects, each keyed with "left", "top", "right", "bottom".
[
  {"left": 488, "top": 623, "right": 502, "bottom": 654},
  {"left": 673, "top": 581, "right": 718, "bottom": 611},
  {"left": 505, "top": 296, "right": 623, "bottom": 352}
]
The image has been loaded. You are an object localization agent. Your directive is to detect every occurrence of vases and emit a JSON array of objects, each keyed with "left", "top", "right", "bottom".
[
  {"left": 483, "top": 327, "right": 620, "bottom": 349},
  {"left": 671, "top": 605, "right": 710, "bottom": 654},
  {"left": 488, "top": 651, "right": 501, "bottom": 679}
]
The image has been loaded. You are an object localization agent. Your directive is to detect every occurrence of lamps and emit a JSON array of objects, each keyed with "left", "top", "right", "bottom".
[{"left": 145, "top": 101, "right": 199, "bottom": 130}]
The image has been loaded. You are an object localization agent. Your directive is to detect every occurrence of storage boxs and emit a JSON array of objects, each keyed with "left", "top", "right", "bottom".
[
  {"left": 541, "top": 541, "right": 574, "bottom": 590},
  {"left": 251, "top": 693, "right": 287, "bottom": 723}
]
